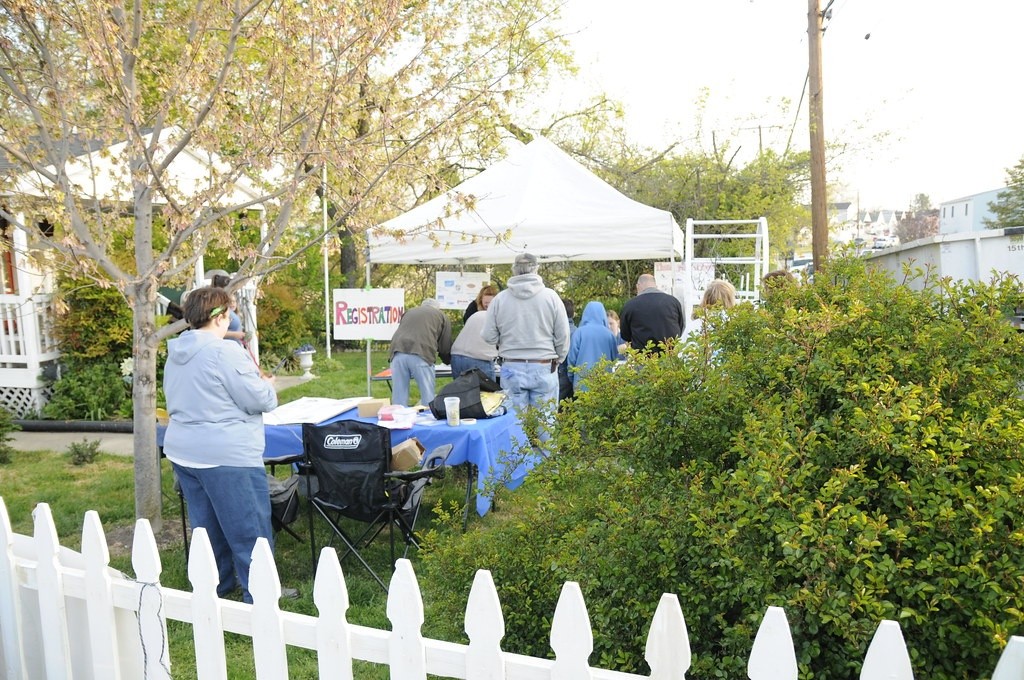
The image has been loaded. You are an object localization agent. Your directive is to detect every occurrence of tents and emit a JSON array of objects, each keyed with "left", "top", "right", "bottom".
[{"left": 365, "top": 135, "right": 684, "bottom": 397}]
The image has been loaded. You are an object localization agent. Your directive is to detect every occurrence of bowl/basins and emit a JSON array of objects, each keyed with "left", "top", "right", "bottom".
[{"left": 392, "top": 408, "right": 416, "bottom": 423}]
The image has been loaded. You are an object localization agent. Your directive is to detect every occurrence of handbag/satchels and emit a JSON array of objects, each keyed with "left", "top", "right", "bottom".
[{"left": 428, "top": 367, "right": 508, "bottom": 421}]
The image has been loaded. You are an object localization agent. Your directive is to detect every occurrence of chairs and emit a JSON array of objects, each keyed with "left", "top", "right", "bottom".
[
  {"left": 172, "top": 461, "right": 306, "bottom": 563},
  {"left": 296, "top": 420, "right": 454, "bottom": 595}
]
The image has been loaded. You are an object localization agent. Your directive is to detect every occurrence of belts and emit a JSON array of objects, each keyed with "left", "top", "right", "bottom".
[{"left": 504, "top": 359, "right": 552, "bottom": 364}]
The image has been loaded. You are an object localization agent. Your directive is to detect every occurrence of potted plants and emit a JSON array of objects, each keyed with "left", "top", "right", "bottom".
[{"left": 294, "top": 343, "right": 317, "bottom": 377}]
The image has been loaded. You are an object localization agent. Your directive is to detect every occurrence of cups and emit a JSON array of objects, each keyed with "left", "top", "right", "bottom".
[{"left": 444, "top": 397, "right": 460, "bottom": 426}]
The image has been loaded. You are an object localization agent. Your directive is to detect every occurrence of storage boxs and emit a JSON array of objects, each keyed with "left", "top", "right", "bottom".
[
  {"left": 391, "top": 437, "right": 426, "bottom": 471},
  {"left": 359, "top": 398, "right": 390, "bottom": 417}
]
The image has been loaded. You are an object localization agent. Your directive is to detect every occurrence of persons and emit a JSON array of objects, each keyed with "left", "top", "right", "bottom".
[
  {"left": 619, "top": 274, "right": 684, "bottom": 358},
  {"left": 761, "top": 271, "right": 794, "bottom": 299},
  {"left": 450, "top": 253, "right": 625, "bottom": 447},
  {"left": 681, "top": 278, "right": 736, "bottom": 341},
  {"left": 389, "top": 298, "right": 452, "bottom": 408},
  {"left": 163, "top": 275, "right": 300, "bottom": 604}
]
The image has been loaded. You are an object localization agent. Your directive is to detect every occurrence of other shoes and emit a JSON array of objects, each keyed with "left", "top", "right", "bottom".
[
  {"left": 282, "top": 588, "right": 301, "bottom": 600},
  {"left": 240, "top": 634, "right": 253, "bottom": 644}
]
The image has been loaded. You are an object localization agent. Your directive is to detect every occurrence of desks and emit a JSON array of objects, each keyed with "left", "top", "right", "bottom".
[
  {"left": 367, "top": 364, "right": 500, "bottom": 393},
  {"left": 154, "top": 396, "right": 539, "bottom": 535}
]
